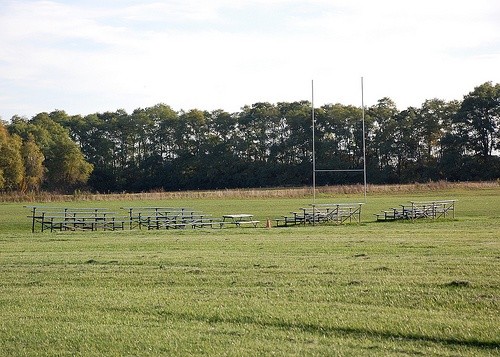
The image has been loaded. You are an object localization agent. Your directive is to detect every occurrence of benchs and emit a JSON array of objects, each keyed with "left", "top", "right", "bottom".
[{"left": 20, "top": 196, "right": 459, "bottom": 232}]
